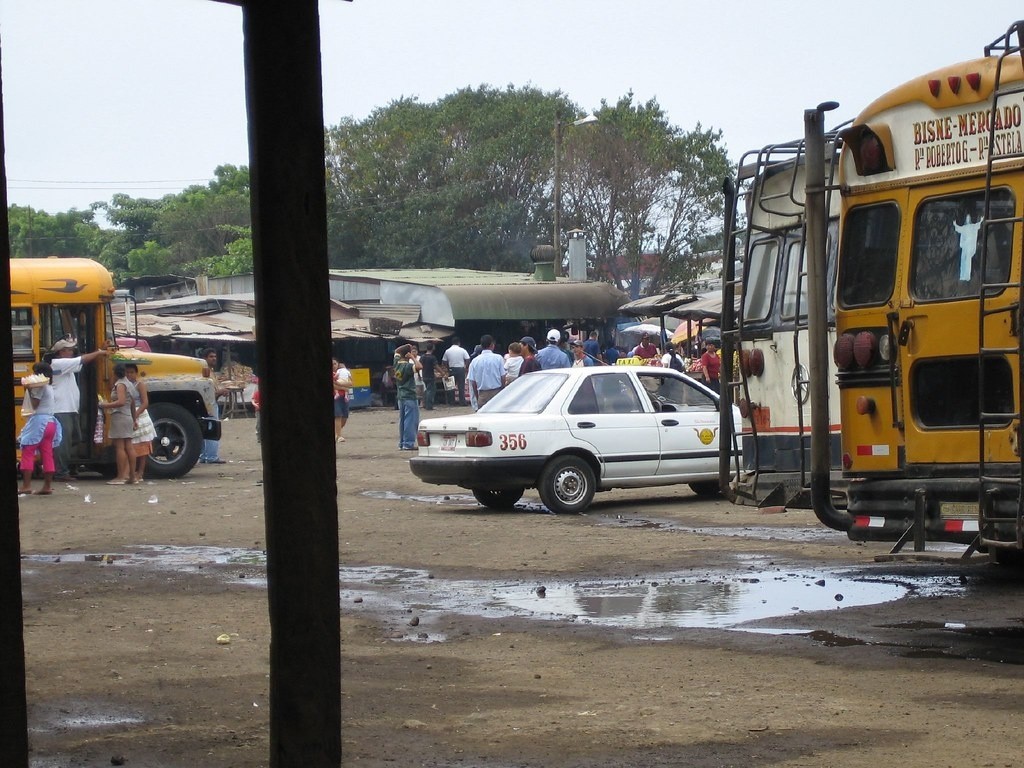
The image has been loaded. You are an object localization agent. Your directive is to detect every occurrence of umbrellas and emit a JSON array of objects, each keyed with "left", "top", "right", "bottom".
[{"left": 615, "top": 292, "right": 743, "bottom": 364}]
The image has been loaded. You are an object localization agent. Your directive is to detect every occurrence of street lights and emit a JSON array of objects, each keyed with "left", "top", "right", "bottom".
[{"left": 554, "top": 114, "right": 601, "bottom": 274}]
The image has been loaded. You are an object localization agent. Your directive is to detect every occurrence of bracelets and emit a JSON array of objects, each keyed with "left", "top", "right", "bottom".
[{"left": 441, "top": 372, "right": 447, "bottom": 377}]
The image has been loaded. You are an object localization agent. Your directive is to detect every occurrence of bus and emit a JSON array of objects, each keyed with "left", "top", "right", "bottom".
[
  {"left": 8, "top": 257, "right": 222, "bottom": 478},
  {"left": 718, "top": 22, "right": 1024, "bottom": 568}
]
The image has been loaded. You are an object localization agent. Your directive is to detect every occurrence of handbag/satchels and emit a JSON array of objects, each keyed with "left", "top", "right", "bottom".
[
  {"left": 94, "top": 406, "right": 106, "bottom": 444},
  {"left": 21, "top": 388, "right": 35, "bottom": 417},
  {"left": 442, "top": 376, "right": 455, "bottom": 391}
]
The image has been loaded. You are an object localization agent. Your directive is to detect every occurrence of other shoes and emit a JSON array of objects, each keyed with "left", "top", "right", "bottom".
[
  {"left": 207, "top": 458, "right": 227, "bottom": 463},
  {"left": 199, "top": 459, "right": 205, "bottom": 463},
  {"left": 337, "top": 437, "right": 345, "bottom": 442}
]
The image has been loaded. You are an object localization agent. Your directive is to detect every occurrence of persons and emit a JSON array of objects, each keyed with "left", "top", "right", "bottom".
[
  {"left": 17, "top": 363, "right": 56, "bottom": 496},
  {"left": 583, "top": 331, "right": 657, "bottom": 365},
  {"left": 601, "top": 376, "right": 620, "bottom": 414},
  {"left": 251, "top": 390, "right": 261, "bottom": 445},
  {"left": 331, "top": 359, "right": 352, "bottom": 442},
  {"left": 97, "top": 363, "right": 157, "bottom": 485},
  {"left": 49, "top": 337, "right": 108, "bottom": 480},
  {"left": 660, "top": 342, "right": 686, "bottom": 376},
  {"left": 394, "top": 343, "right": 423, "bottom": 451},
  {"left": 408, "top": 337, "right": 471, "bottom": 411},
  {"left": 468, "top": 329, "right": 593, "bottom": 413},
  {"left": 701, "top": 342, "right": 720, "bottom": 395},
  {"left": 198, "top": 348, "right": 227, "bottom": 463}
]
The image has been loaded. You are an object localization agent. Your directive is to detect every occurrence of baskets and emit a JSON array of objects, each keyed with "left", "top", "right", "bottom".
[{"left": 21, "top": 377, "right": 50, "bottom": 387}]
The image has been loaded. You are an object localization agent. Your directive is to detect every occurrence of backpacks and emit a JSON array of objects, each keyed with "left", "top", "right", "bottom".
[{"left": 669, "top": 352, "right": 683, "bottom": 372}]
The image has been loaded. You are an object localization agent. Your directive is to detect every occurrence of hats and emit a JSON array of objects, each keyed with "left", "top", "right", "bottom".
[
  {"left": 470, "top": 345, "right": 482, "bottom": 356},
  {"left": 568, "top": 340, "right": 584, "bottom": 346},
  {"left": 520, "top": 336, "right": 538, "bottom": 354},
  {"left": 547, "top": 329, "right": 560, "bottom": 342},
  {"left": 50, "top": 337, "right": 77, "bottom": 351}
]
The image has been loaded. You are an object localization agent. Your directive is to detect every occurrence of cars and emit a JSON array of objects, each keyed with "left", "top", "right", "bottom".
[{"left": 409, "top": 356, "right": 743, "bottom": 514}]
